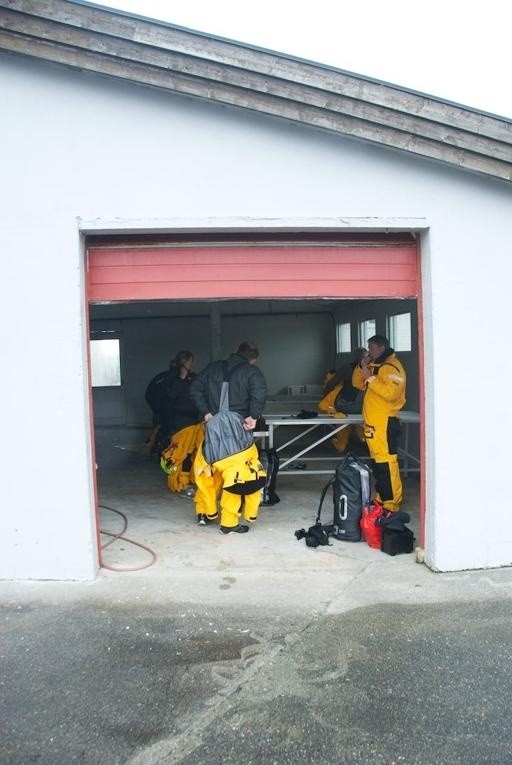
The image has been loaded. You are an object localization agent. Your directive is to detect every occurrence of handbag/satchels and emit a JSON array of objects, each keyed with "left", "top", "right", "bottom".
[
  {"left": 331, "top": 457, "right": 372, "bottom": 543},
  {"left": 259, "top": 448, "right": 280, "bottom": 507}
]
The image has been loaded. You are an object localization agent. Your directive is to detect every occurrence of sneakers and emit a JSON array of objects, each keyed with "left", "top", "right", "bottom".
[
  {"left": 198, "top": 513, "right": 208, "bottom": 525},
  {"left": 220, "top": 524, "right": 249, "bottom": 535},
  {"left": 179, "top": 488, "right": 196, "bottom": 500}
]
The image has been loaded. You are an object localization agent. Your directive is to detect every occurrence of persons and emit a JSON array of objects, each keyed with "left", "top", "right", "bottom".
[
  {"left": 145, "top": 359, "right": 176, "bottom": 445},
  {"left": 317, "top": 347, "right": 369, "bottom": 452},
  {"left": 189, "top": 341, "right": 266, "bottom": 534},
  {"left": 351, "top": 335, "right": 407, "bottom": 512},
  {"left": 164, "top": 351, "right": 203, "bottom": 500}
]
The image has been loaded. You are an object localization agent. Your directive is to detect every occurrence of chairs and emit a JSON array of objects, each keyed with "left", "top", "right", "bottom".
[{"left": 288, "top": 385, "right": 304, "bottom": 395}]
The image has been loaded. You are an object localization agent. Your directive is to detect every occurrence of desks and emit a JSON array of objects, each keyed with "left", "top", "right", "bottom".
[{"left": 254, "top": 395, "right": 419, "bottom": 479}]
